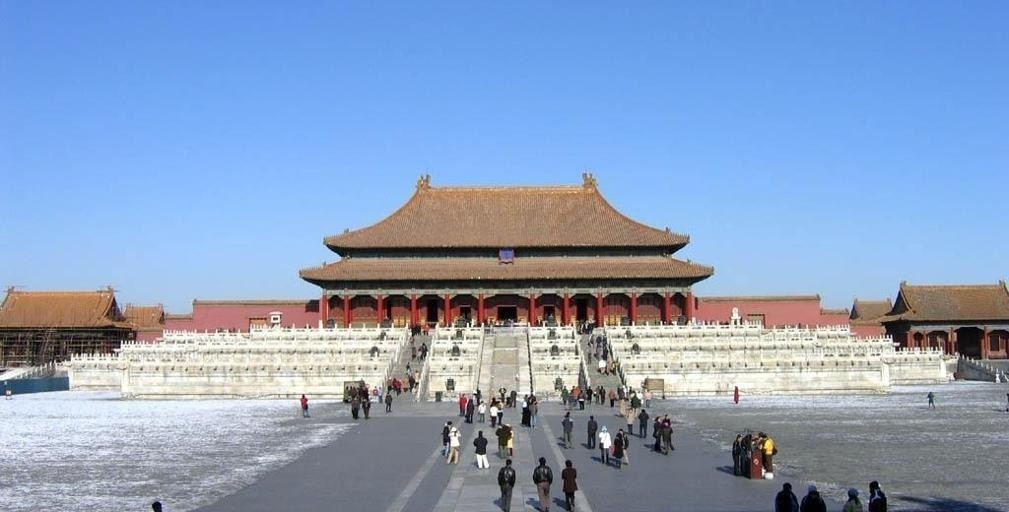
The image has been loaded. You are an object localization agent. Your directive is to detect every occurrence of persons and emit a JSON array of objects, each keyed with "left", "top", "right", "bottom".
[
  {"left": 344, "top": 385, "right": 370, "bottom": 419},
  {"left": 385, "top": 392, "right": 392, "bottom": 412},
  {"left": 731, "top": 431, "right": 775, "bottom": 479},
  {"left": 387, "top": 362, "right": 421, "bottom": 396},
  {"left": 533, "top": 455, "right": 553, "bottom": 511},
  {"left": 842, "top": 488, "right": 864, "bottom": 512},
  {"left": 151, "top": 501, "right": 163, "bottom": 512},
  {"left": 489, "top": 387, "right": 517, "bottom": 427},
  {"left": 562, "top": 412, "right": 629, "bottom": 469},
  {"left": 595, "top": 384, "right": 639, "bottom": 409},
  {"left": 373, "top": 385, "right": 382, "bottom": 402},
  {"left": 301, "top": 394, "right": 310, "bottom": 417},
  {"left": 927, "top": 391, "right": 936, "bottom": 408},
  {"left": 626, "top": 408, "right": 675, "bottom": 455},
  {"left": 800, "top": 484, "right": 827, "bottom": 512},
  {"left": 775, "top": 482, "right": 800, "bottom": 512},
  {"left": 561, "top": 384, "right": 593, "bottom": 410},
  {"left": 443, "top": 420, "right": 513, "bottom": 468},
  {"left": 410, "top": 321, "right": 429, "bottom": 337},
  {"left": 412, "top": 343, "right": 427, "bottom": 360},
  {"left": 867, "top": 481, "right": 887, "bottom": 511},
  {"left": 587, "top": 334, "right": 616, "bottom": 376},
  {"left": 459, "top": 389, "right": 486, "bottom": 423},
  {"left": 498, "top": 459, "right": 515, "bottom": 510},
  {"left": 561, "top": 458, "right": 579, "bottom": 511},
  {"left": 522, "top": 393, "right": 538, "bottom": 428}
]
